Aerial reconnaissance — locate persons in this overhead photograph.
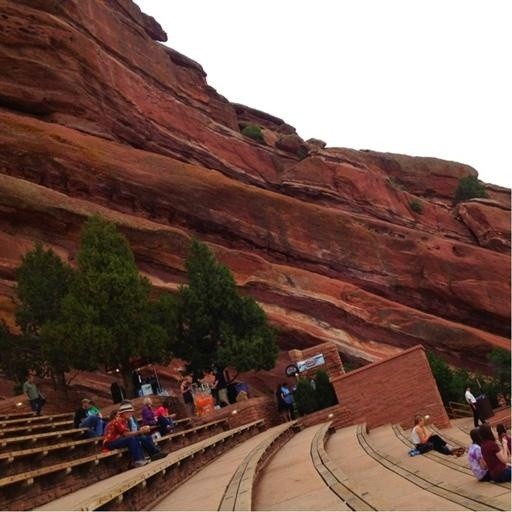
[181,378,194,417]
[22,374,46,417]
[464,386,489,428]
[412,416,512,484]
[281,382,298,421]
[73,396,178,468]
[275,383,295,423]
[211,366,231,407]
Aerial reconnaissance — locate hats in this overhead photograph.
[118,403,135,414]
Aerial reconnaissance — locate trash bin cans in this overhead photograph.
[110,382,122,404]
[475,394,494,420]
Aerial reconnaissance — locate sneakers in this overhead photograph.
[455,445,464,457]
[128,452,169,469]
